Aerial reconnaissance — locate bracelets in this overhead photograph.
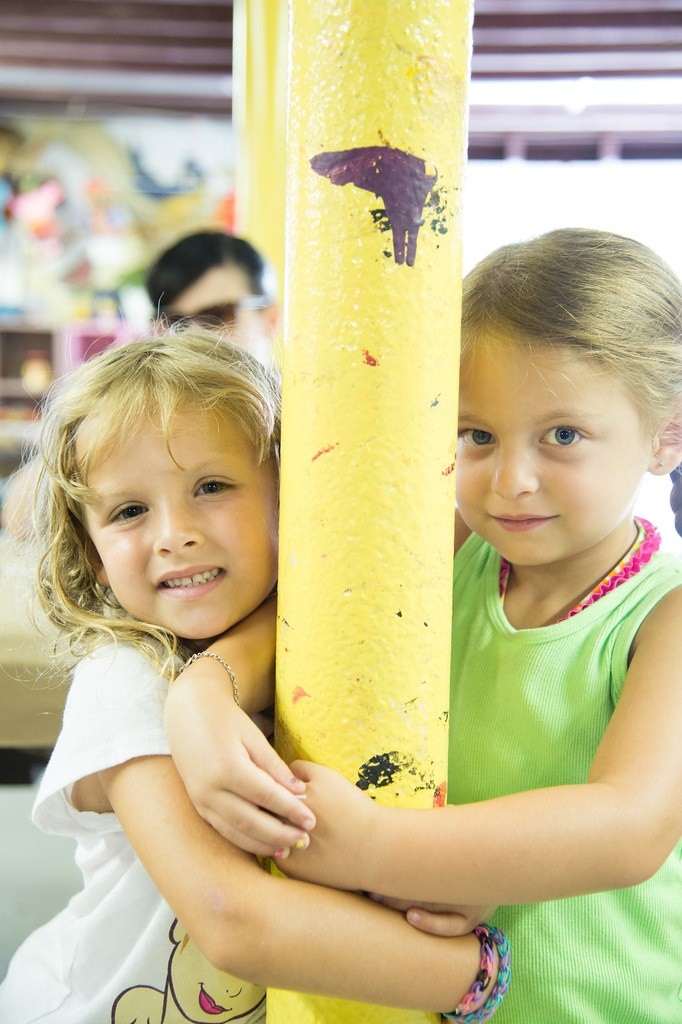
[443,924,511,1024]
[178,650,240,705]
[455,926,495,1015]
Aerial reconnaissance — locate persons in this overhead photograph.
[144,231,279,368]
[161,226,682,1024]
[1,327,512,1022]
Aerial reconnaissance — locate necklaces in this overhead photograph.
[499,516,663,622]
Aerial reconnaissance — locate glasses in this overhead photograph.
[160,296,269,333]
